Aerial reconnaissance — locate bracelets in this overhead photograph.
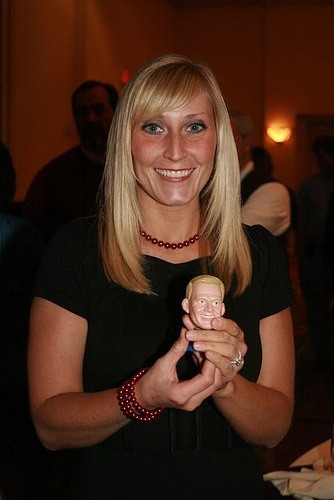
[118,367,166,422]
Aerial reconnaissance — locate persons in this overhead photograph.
[26,54,296,500]
[181,274,226,330]
[0,79,334,434]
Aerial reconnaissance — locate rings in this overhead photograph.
[230,350,244,366]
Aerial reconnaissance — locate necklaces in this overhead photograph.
[139,227,204,250]
